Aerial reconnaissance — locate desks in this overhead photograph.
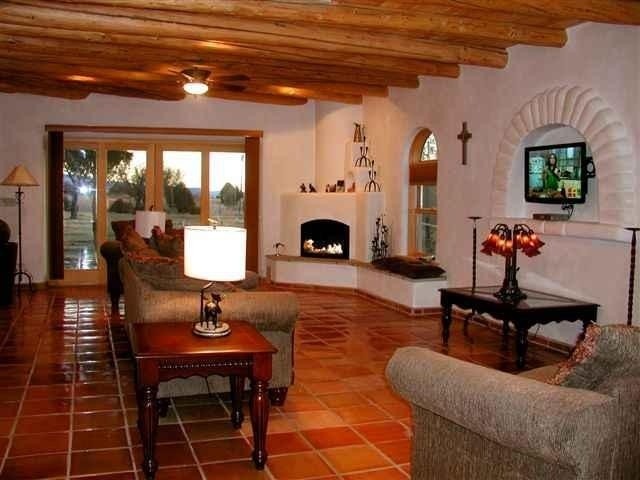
[125,319,279,479]
[436,284,601,372]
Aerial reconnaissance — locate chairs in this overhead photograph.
[119,256,303,418]
[382,341,638,480]
[98,238,153,317]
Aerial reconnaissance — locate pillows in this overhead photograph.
[540,319,640,402]
[111,219,239,293]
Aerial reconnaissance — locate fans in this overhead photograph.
[163,68,251,95]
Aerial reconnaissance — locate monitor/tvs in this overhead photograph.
[524,141,588,204]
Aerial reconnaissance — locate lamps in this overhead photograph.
[480,223,546,306]
[181,80,209,95]
[1,162,41,296]
[182,224,248,339]
[135,204,167,241]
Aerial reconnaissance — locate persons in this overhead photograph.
[539,152,563,199]
